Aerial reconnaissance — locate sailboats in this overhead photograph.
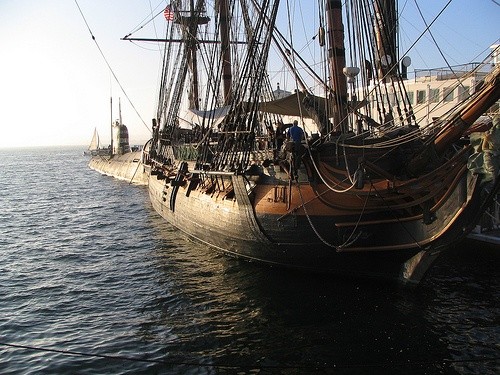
[83,128,103,156]
[121,0,500,281]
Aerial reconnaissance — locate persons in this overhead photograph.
[353,122,401,136]
[266,122,285,139]
[286,120,306,145]
[383,109,393,126]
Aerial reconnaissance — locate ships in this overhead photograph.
[88,97,150,185]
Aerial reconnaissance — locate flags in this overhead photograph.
[163,6,175,21]
